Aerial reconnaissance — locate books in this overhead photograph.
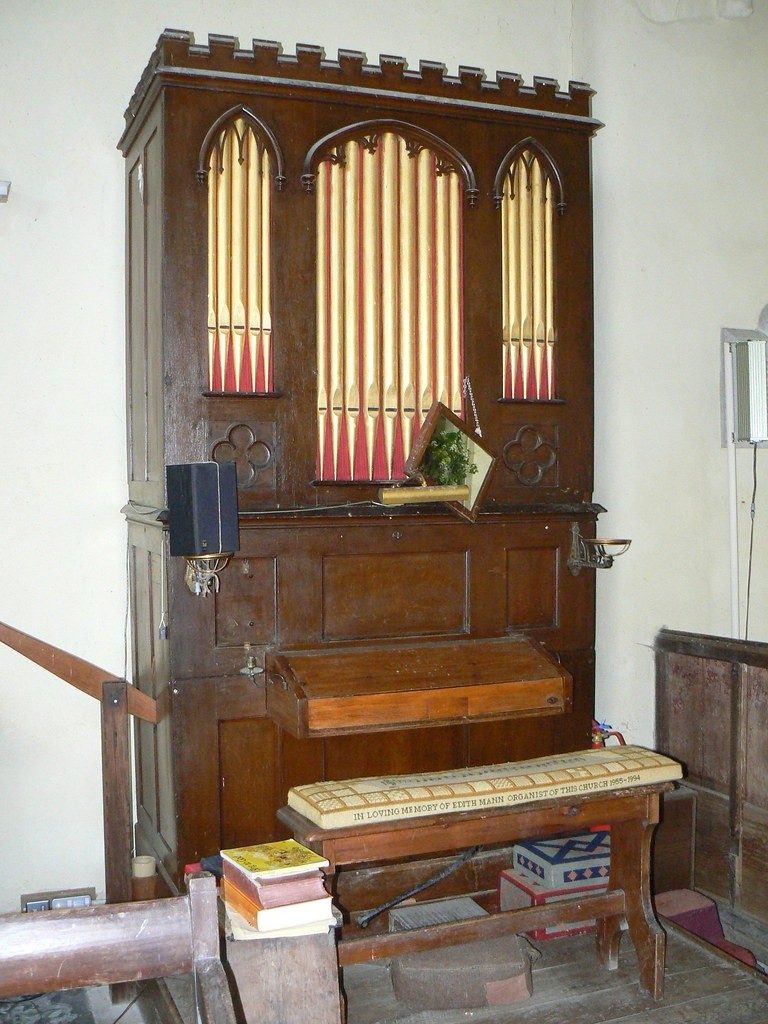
[184,837,338,941]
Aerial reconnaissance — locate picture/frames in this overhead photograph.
[402,401,501,524]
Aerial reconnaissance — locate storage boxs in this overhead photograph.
[497,869,609,940]
[513,831,611,889]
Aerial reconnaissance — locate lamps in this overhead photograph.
[378,472,469,505]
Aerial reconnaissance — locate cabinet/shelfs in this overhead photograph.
[116,25,604,942]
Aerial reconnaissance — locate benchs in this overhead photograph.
[276,745,683,1024]
